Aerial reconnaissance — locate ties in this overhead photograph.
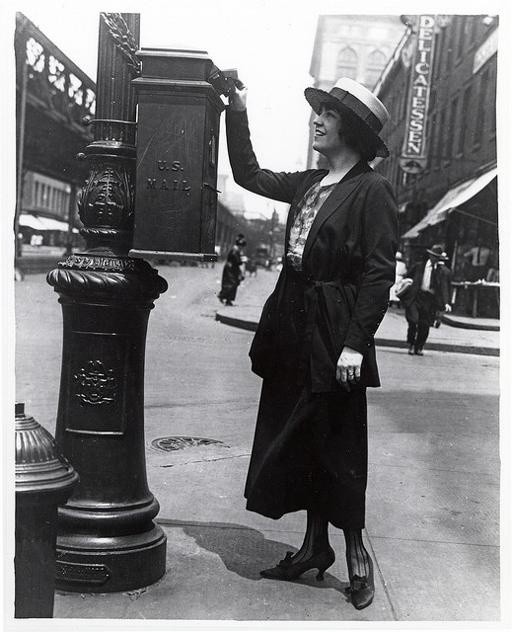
[430,263,437,288]
[477,246,481,264]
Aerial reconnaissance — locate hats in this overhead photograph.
[304,77,390,158]
[426,245,446,257]
[395,251,402,259]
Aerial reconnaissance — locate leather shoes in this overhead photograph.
[408,344,424,356]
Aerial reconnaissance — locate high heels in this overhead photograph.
[259,545,335,581]
[346,546,375,610]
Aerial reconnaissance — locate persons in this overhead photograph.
[224,76,400,610]
[401,245,452,356]
[430,252,452,328]
[388,252,407,308]
[217,234,247,306]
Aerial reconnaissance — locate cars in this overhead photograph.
[252,245,272,270]
